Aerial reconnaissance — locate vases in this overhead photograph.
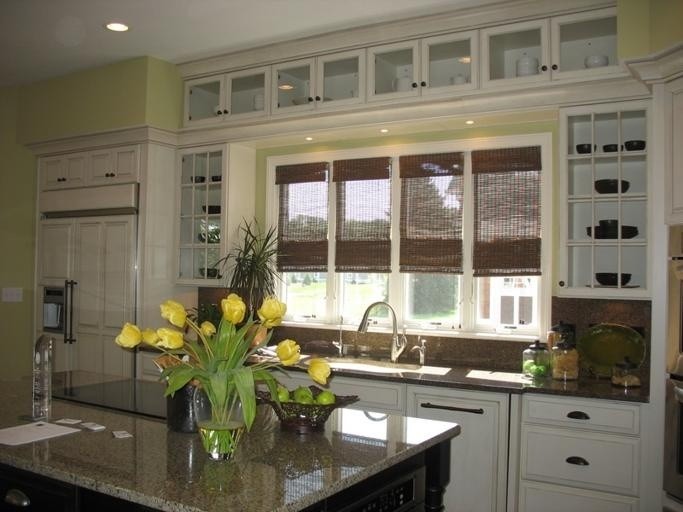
[193,384,251,462]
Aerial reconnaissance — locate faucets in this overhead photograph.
[358,302,407,363]
[411,340,426,365]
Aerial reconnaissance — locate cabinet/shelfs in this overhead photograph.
[254,367,509,512]
[520,393,662,512]
[36,146,140,190]
[560,100,653,300]
[35,214,139,411]
[182,7,620,130]
[175,144,255,287]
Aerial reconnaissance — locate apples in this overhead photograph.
[271,386,337,408]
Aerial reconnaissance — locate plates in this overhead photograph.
[586,226,636,238]
[254,94,263,107]
[586,283,639,290]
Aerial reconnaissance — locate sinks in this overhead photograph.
[304,357,422,373]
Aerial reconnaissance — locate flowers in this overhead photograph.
[116,292,331,453]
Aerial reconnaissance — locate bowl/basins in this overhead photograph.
[603,143,622,152]
[210,174,223,182]
[202,205,223,217]
[190,177,206,182]
[595,272,632,288]
[575,143,596,153]
[597,219,617,227]
[595,178,630,193]
[625,140,646,150]
[584,55,608,70]
[516,56,539,76]
